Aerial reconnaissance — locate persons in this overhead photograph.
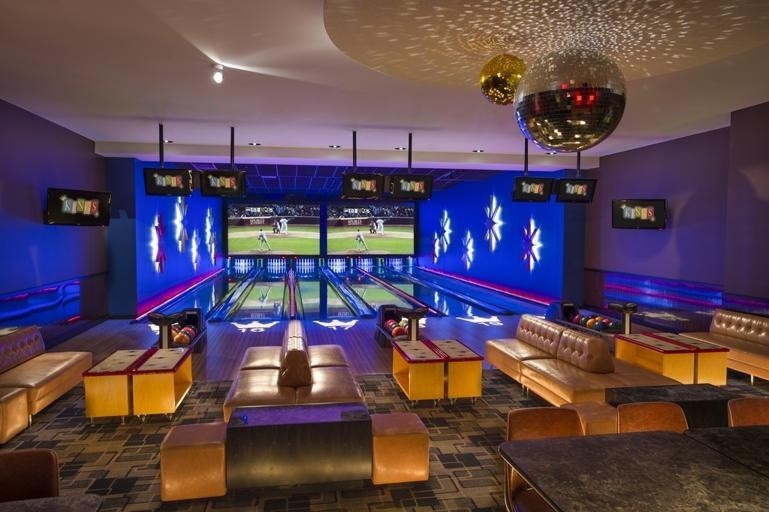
[258,228,272,250]
[272,218,288,236]
[355,229,368,251]
[369,218,384,236]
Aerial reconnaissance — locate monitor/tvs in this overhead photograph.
[389,174,433,201]
[513,176,553,203]
[328,199,415,255]
[557,179,596,202]
[224,201,321,257]
[341,173,382,199]
[144,167,191,196]
[200,169,245,197]
[46,188,111,226]
[611,198,665,229]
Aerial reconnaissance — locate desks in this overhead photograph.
[498,425,753,511]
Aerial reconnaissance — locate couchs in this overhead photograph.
[370,413,432,486]
[238,320,295,372]
[610,335,685,386]
[223,336,296,421]
[485,313,566,385]
[294,339,367,405]
[0,386,31,445]
[679,309,769,389]
[519,329,629,408]
[162,423,226,505]
[297,321,352,366]
[1,327,94,426]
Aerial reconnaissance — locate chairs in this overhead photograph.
[726,393,769,427]
[506,407,584,512]
[613,402,690,435]
[0,450,59,501]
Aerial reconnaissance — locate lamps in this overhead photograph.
[212,64,225,86]
[480,54,524,106]
[514,51,627,156]
